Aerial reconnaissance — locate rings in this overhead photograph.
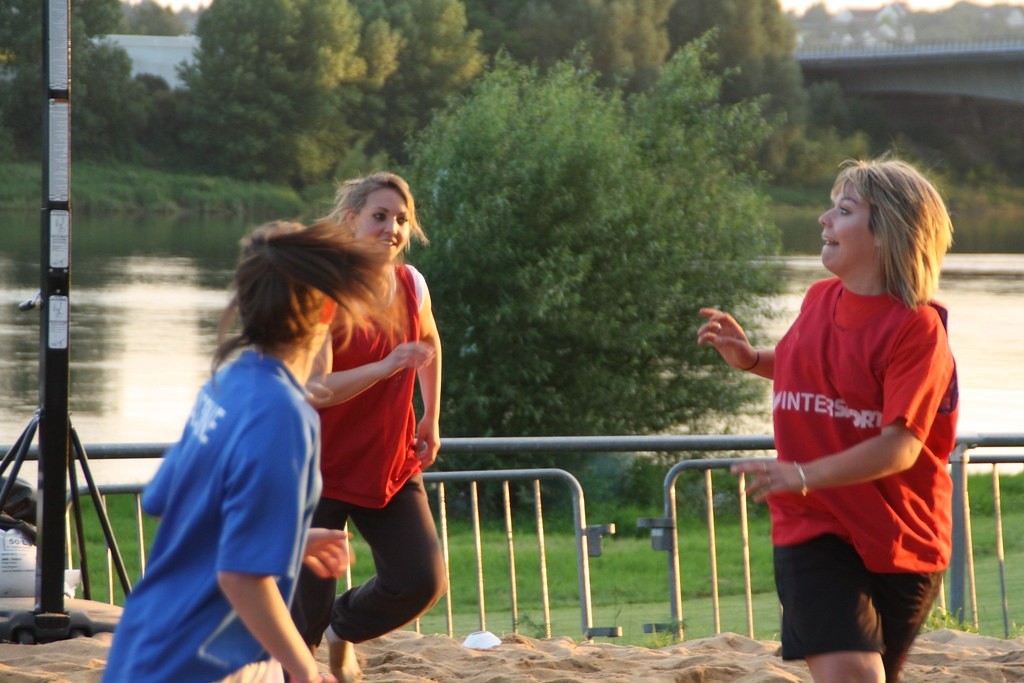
[763,462,768,472]
[766,474,771,486]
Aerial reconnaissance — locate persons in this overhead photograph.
[101,219,405,683]
[699,149,958,683]
[280,171,450,683]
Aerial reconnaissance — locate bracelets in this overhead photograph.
[792,459,807,498]
[741,351,760,371]
